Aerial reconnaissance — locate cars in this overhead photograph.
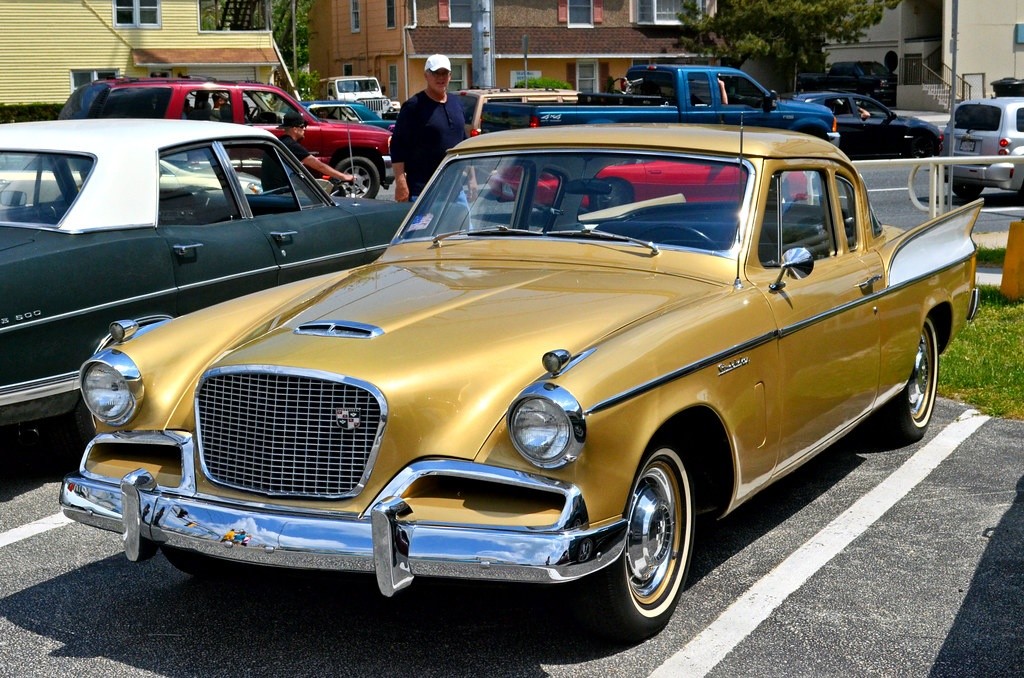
[0,152,263,213]
[781,87,944,158]
[0,118,478,466]
[58,123,986,653]
[275,98,399,130]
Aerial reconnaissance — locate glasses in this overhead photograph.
[295,125,306,129]
[429,69,449,77]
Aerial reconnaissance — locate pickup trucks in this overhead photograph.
[480,63,840,151]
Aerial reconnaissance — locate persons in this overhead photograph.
[261,112,358,195]
[717,78,728,105]
[212,93,250,124]
[845,99,870,119]
[390,54,478,231]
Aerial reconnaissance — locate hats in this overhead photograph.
[424,54,451,72]
[276,111,304,129]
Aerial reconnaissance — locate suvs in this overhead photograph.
[448,83,581,139]
[58,66,396,201]
[318,73,400,120]
[938,96,1024,205]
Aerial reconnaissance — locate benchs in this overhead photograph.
[594,201,849,262]
